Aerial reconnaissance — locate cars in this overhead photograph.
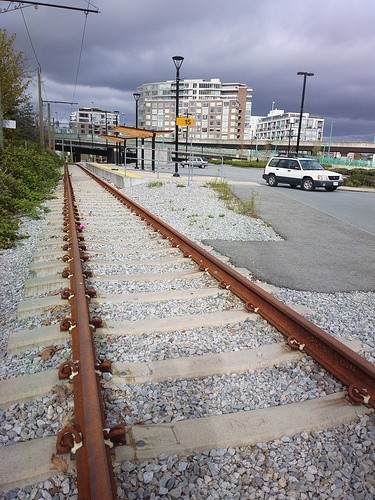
[261,156,345,192]
[180,156,209,169]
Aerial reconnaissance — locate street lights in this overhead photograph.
[133,93,142,170]
[114,110,121,165]
[91,101,95,142]
[172,55,186,176]
[122,113,125,126]
[296,71,315,153]
